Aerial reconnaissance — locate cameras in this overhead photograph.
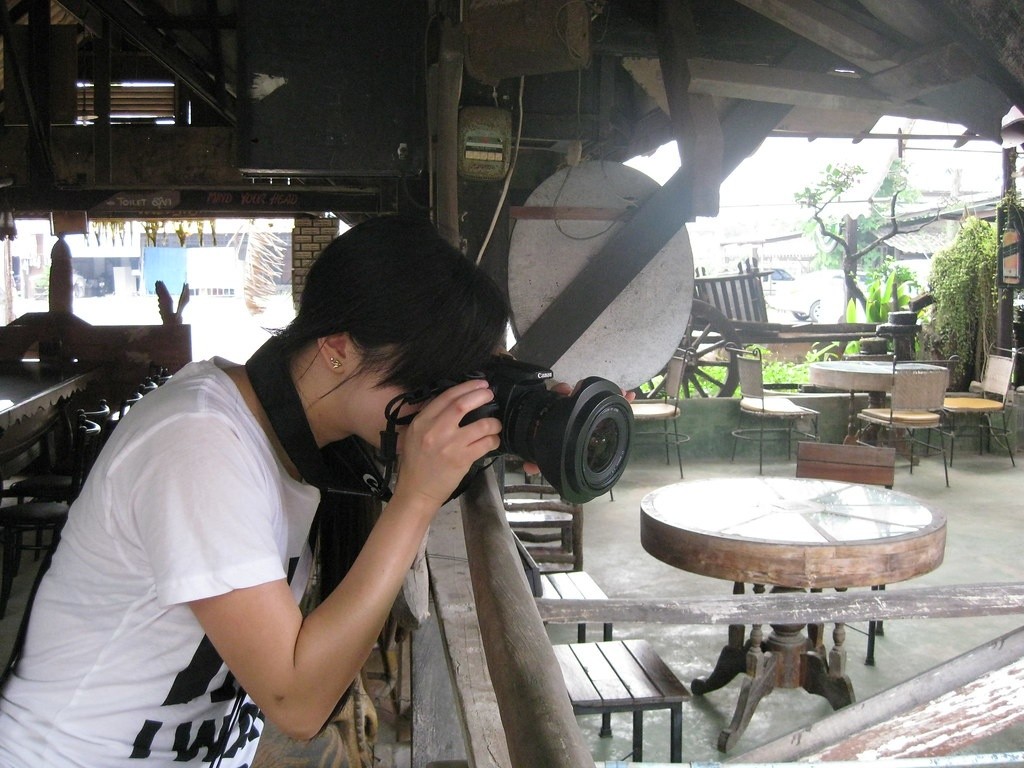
[423,355,635,507]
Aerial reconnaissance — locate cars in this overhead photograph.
[770,268,887,324]
[762,267,799,302]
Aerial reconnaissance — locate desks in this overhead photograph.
[1,358,100,431]
[638,478,945,752]
[808,360,934,446]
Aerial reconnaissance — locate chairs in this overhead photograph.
[1,364,173,621]
[924,342,1017,465]
[724,343,819,475]
[493,450,691,763]
[623,347,697,478]
[856,355,950,488]
[790,439,904,665]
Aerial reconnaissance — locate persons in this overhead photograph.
[1,217,637,768]
